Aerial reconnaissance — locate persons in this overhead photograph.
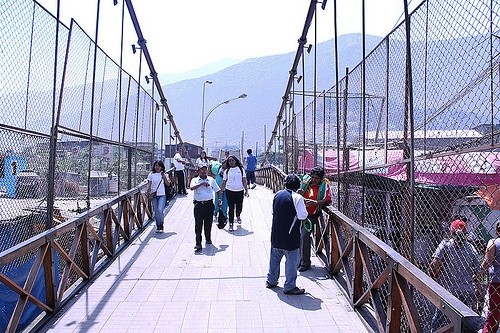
[245,149,257,189]
[190,164,227,250]
[220,155,249,231]
[195,151,235,222]
[172,147,189,195]
[145,160,171,233]
[266,173,310,294]
[296,166,332,272]
[356,210,500,333]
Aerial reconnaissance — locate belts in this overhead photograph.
[196,199,212,204]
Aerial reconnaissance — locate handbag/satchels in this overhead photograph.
[148,191,157,201]
[217,208,227,229]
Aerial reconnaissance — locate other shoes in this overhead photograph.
[178,191,182,195]
[251,184,256,189]
[206,239,212,244]
[229,223,233,231]
[183,191,188,195]
[297,260,311,272]
[283,287,305,295]
[247,185,250,189]
[266,280,279,288]
[194,245,202,249]
[237,217,242,224]
[155,226,163,233]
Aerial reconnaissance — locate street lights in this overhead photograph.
[202,94,248,150]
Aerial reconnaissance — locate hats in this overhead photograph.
[310,167,324,180]
[285,174,301,188]
[196,163,207,171]
[450,219,468,233]
